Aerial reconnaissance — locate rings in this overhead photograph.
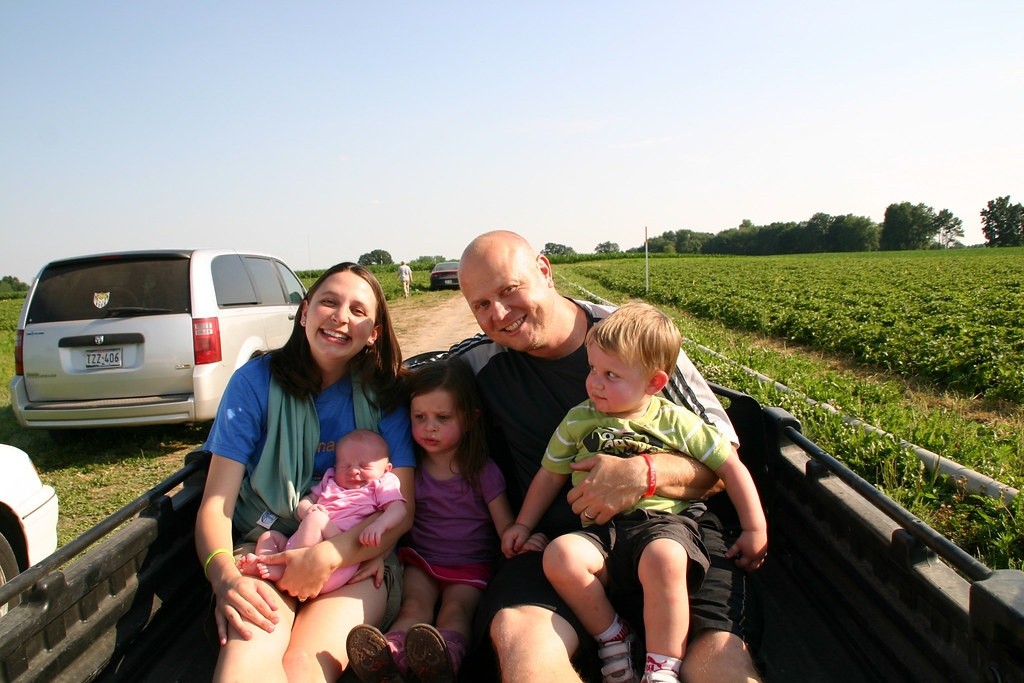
[584,510,594,521]
[298,596,307,602]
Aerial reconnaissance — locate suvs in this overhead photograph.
[9,246,310,437]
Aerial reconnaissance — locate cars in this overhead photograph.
[429,262,461,289]
[0,444,60,621]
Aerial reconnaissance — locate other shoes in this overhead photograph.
[404,623,453,683]
[346,624,409,683]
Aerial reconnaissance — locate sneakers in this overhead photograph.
[641,672,681,683]
[598,630,640,683]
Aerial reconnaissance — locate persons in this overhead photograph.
[194,262,415,683]
[397,261,412,298]
[501,303,768,683]
[402,229,763,683]
[236,430,408,595]
[346,358,548,683]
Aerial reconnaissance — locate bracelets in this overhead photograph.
[641,454,656,498]
[204,548,235,582]
